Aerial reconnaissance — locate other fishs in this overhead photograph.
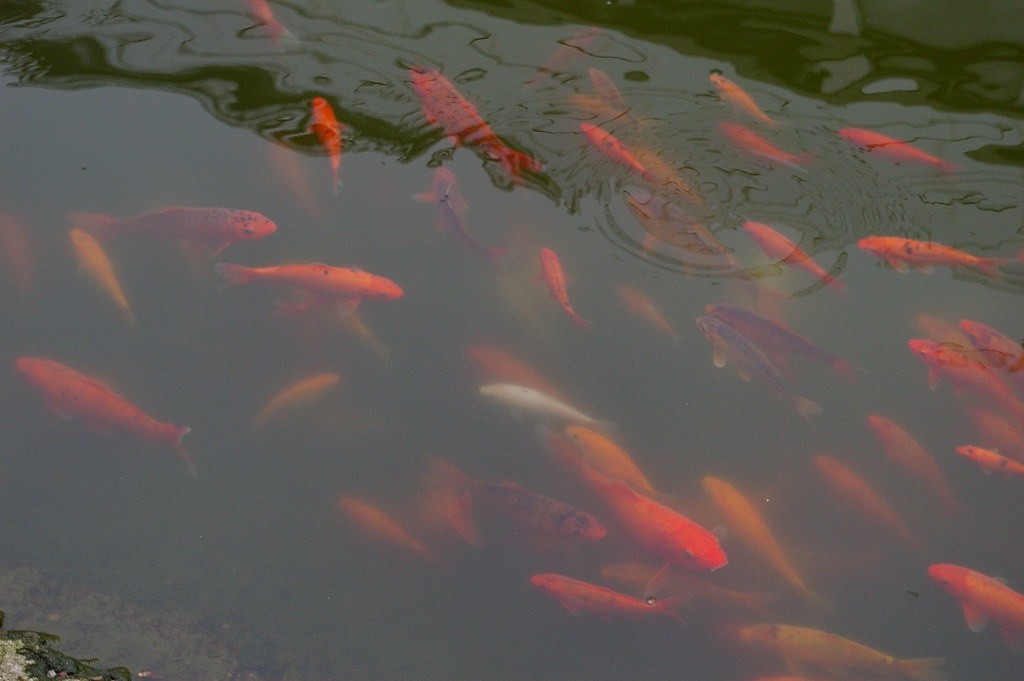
[1,2,1023,680]
